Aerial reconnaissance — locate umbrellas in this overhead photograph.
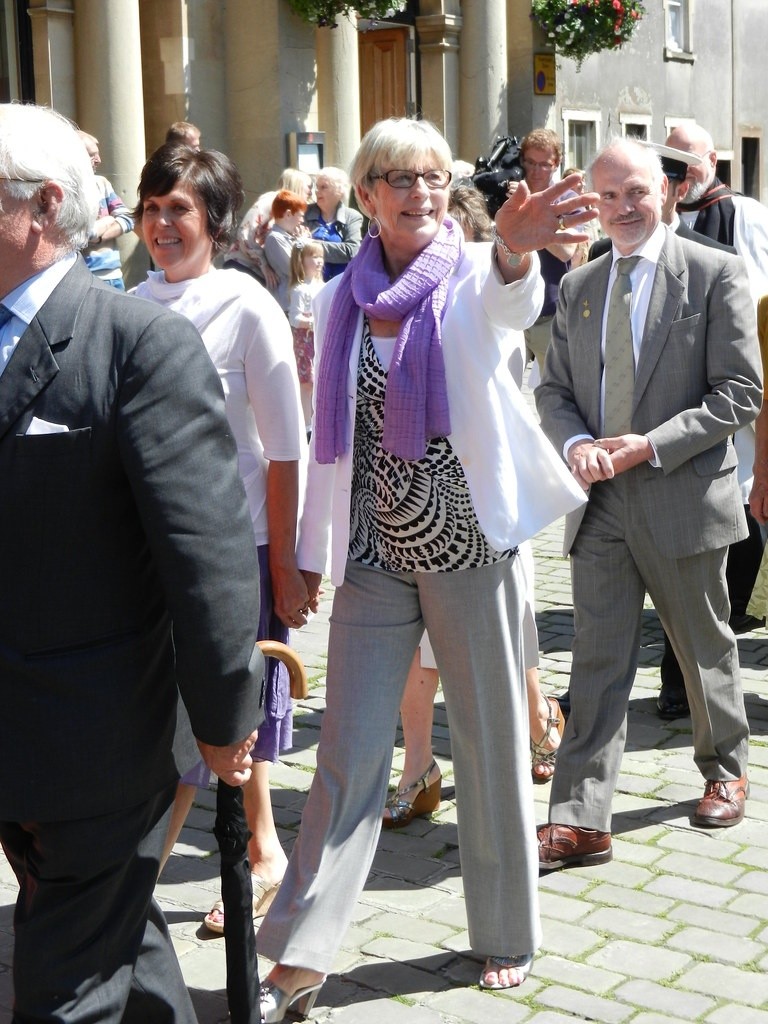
[213,637,307,1024]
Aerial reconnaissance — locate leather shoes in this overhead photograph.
[538,825,612,869]
[730,610,753,628]
[694,777,749,826]
[658,690,690,719]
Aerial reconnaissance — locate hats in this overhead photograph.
[639,140,704,181]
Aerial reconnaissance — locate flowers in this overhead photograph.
[530,0,645,74]
[286,0,408,36]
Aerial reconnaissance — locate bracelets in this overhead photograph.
[96,236,102,244]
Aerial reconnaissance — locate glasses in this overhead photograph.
[523,159,558,171]
[365,169,453,189]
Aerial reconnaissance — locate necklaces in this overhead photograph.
[490,226,529,269]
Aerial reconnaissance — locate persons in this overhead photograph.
[748,296,767,528]
[533,135,764,870]
[121,140,310,935]
[556,142,738,725]
[222,167,364,313]
[259,115,600,1024]
[447,160,609,269]
[132,121,202,242]
[665,122,768,629]
[380,184,564,831]
[507,127,591,388]
[288,242,328,444]
[0,102,266,1024]
[75,129,136,293]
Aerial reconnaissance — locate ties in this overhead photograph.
[604,257,643,439]
[0,304,15,329]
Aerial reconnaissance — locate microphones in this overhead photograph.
[501,154,516,169]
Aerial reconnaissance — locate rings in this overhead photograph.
[559,217,565,230]
[299,609,303,613]
[311,597,315,601]
[292,619,295,622]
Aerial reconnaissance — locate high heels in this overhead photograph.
[530,689,564,779]
[479,955,533,988]
[205,876,285,932]
[381,758,442,827]
[259,977,329,1024]
